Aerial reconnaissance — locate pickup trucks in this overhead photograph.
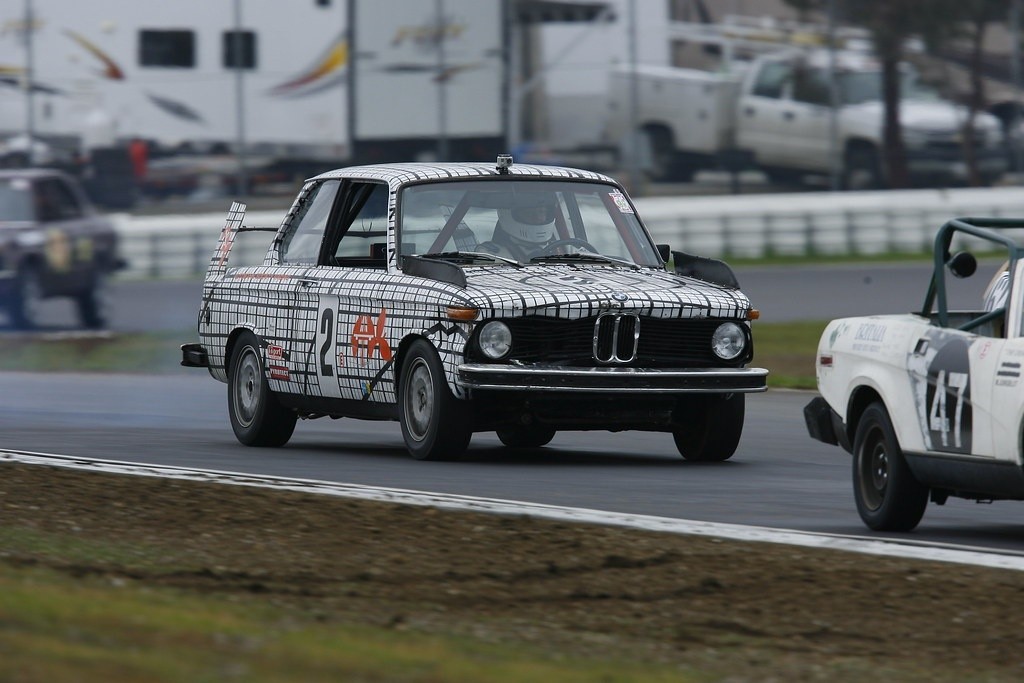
[603,50,1009,191]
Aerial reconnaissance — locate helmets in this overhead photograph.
[497,187,556,244]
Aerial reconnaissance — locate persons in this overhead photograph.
[476,191,591,261]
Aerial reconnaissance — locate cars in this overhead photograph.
[803,218,1024,530]
[0,170,128,328]
[180,155,769,461]
